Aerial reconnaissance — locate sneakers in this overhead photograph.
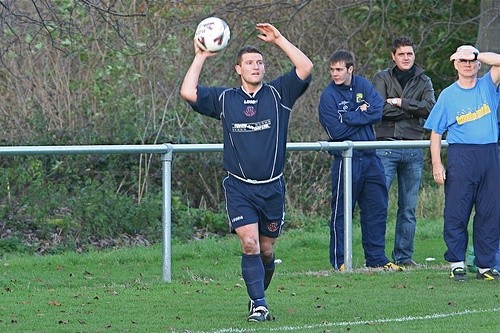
[449,267,467,281]
[383,262,402,272]
[477,269,500,280]
[248,300,255,315]
[248,306,269,322]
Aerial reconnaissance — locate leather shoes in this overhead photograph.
[405,260,427,267]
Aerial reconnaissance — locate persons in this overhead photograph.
[180,23,314,322]
[423,45,500,281]
[318,50,405,274]
[371,36,436,270]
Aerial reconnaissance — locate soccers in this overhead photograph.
[195,16,231,53]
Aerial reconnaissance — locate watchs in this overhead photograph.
[391,97,398,107]
[473,52,479,60]
